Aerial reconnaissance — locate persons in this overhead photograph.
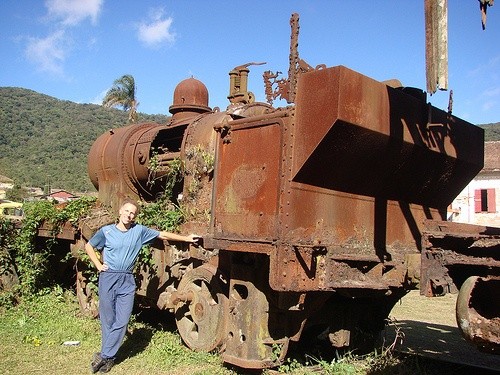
[85,203,203,374]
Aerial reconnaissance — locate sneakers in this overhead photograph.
[90,352,114,373]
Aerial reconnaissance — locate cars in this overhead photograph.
[0,201,23,221]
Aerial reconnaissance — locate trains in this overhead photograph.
[37,0,500,369]
